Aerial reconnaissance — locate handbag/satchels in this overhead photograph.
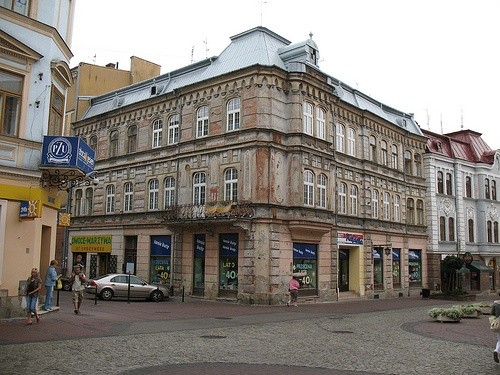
[55,278,62,289]
[488,315,500,332]
[21,295,27,309]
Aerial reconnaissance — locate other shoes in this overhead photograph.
[81,282,88,285]
[24,320,32,325]
[36,316,40,322]
[287,301,290,307]
[74,309,78,314]
[46,308,53,310]
[493,350,499,363]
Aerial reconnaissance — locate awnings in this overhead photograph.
[470,260,491,272]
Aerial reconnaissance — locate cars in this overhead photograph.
[84,273,169,303]
[54,276,73,292]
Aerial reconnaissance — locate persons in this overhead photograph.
[287,276,298,308]
[44,260,62,311]
[25,267,41,326]
[68,255,87,314]
[490,292,500,363]
